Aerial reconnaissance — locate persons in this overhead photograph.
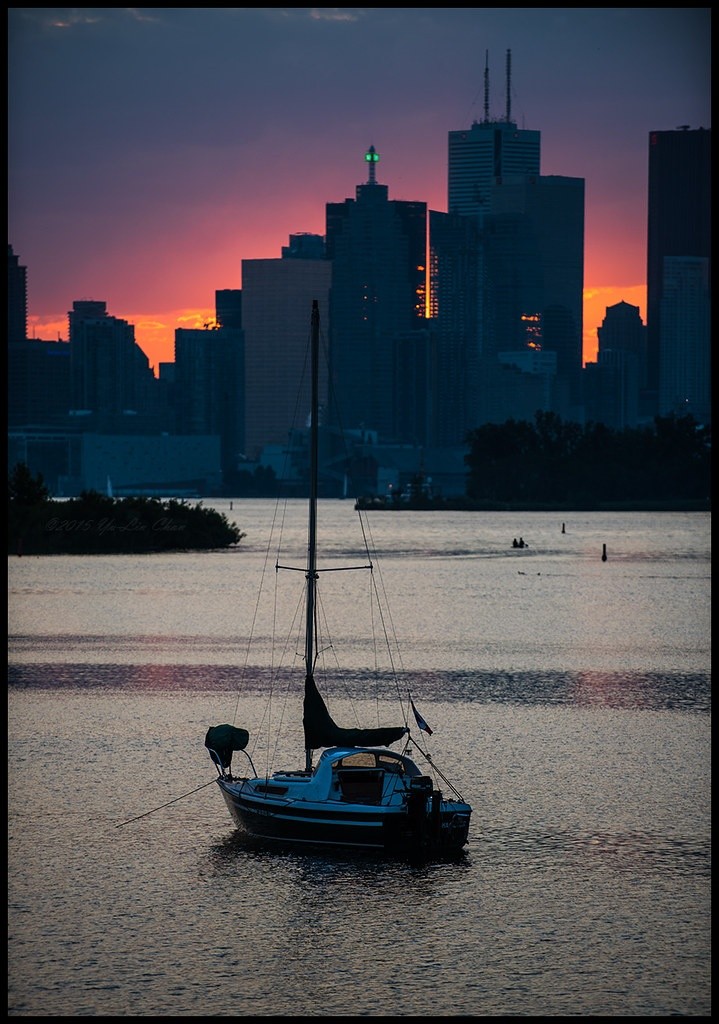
[519,537,525,548]
[513,539,519,548]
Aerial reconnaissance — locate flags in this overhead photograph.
[411,697,433,735]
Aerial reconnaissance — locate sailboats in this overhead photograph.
[201,300,474,855]
